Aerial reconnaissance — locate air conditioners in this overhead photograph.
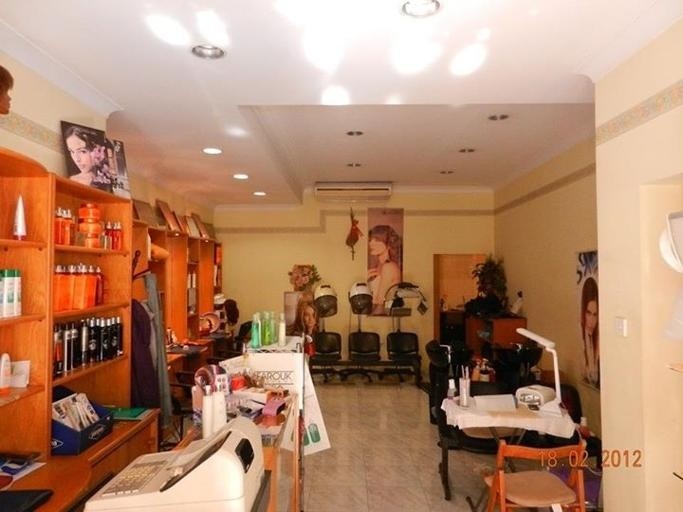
[313,181,392,201]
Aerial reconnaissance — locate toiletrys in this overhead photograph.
[250,311,287,350]
[201,382,228,439]
[0,353,11,399]
[55,194,127,398]
[1,268,23,318]
[12,194,28,239]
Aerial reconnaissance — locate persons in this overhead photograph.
[367,224,400,304]
[63,125,110,192]
[286,289,318,358]
[579,277,598,388]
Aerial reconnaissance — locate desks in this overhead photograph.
[443,394,566,512]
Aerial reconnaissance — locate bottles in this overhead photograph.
[471,358,497,383]
[200,382,227,440]
[250,309,287,350]
[0,202,124,378]
[579,417,591,439]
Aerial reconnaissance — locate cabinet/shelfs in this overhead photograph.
[464,312,526,348]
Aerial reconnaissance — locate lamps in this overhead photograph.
[515,327,562,414]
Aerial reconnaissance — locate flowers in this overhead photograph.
[286,263,322,293]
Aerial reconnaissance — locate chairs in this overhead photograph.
[308,331,422,382]
[483,439,586,512]
